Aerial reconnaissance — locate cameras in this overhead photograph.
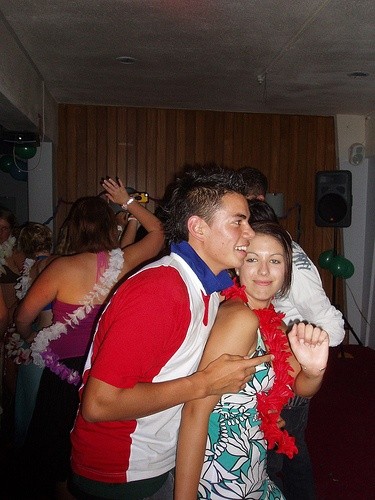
[131,192,149,202]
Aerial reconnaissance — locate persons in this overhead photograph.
[232,166,345,500]
[0,177,166,500]
[66,163,274,500]
[174,222,330,500]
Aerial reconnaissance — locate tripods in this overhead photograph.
[331,229,364,360]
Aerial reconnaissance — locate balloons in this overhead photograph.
[318,249,354,278]
[0,146,37,181]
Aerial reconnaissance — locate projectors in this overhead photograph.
[3,130,41,146]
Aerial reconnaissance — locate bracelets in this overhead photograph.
[300,364,327,371]
[117,225,122,231]
[121,197,134,210]
[128,215,138,221]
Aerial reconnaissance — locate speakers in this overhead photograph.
[348,143,364,166]
[315,170,352,228]
